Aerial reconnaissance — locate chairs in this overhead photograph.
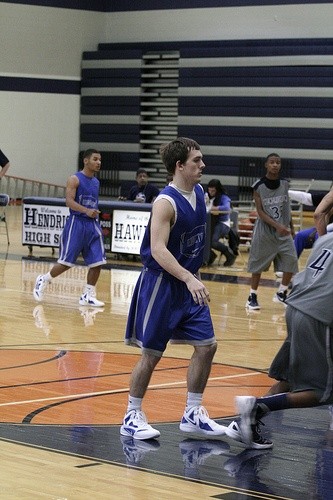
[217,209,247,266]
[0,194,11,245]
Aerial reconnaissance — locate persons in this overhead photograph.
[277,183,333,279]
[224,224,333,450]
[118,135,231,439]
[0,148,10,184]
[117,168,161,204]
[33,148,109,307]
[166,173,174,186]
[198,176,237,268]
[245,149,300,311]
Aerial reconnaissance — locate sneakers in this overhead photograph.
[246,293,261,310]
[272,288,289,307]
[33,274,47,302]
[119,409,161,439]
[235,396,266,445]
[224,419,274,448]
[179,404,228,436]
[79,291,105,306]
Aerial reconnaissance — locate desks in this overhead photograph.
[21,196,230,269]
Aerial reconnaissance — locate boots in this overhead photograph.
[221,247,237,267]
[208,250,217,265]
